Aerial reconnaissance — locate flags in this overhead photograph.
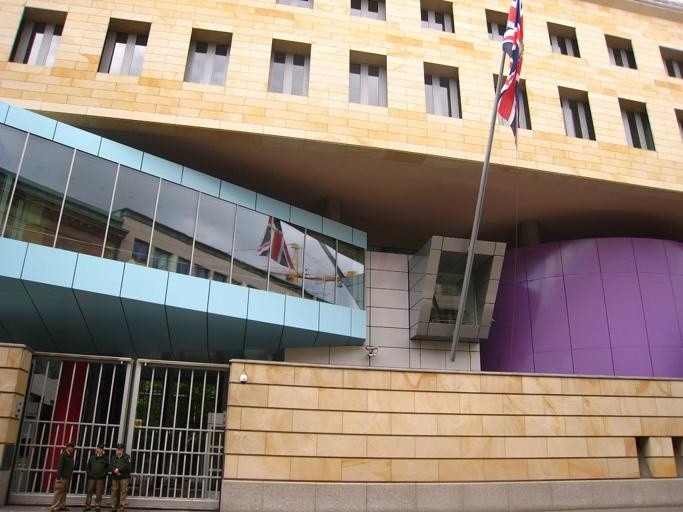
[495,0,524,153]
[256,216,296,270]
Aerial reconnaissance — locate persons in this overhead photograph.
[50,441,74,511]
[82,443,108,511]
[109,443,132,511]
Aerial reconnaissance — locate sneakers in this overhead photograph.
[52,507,101,512]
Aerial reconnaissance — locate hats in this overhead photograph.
[97,444,104,448]
[66,442,76,447]
[116,444,124,449]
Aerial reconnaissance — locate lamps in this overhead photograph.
[240,361,249,384]
